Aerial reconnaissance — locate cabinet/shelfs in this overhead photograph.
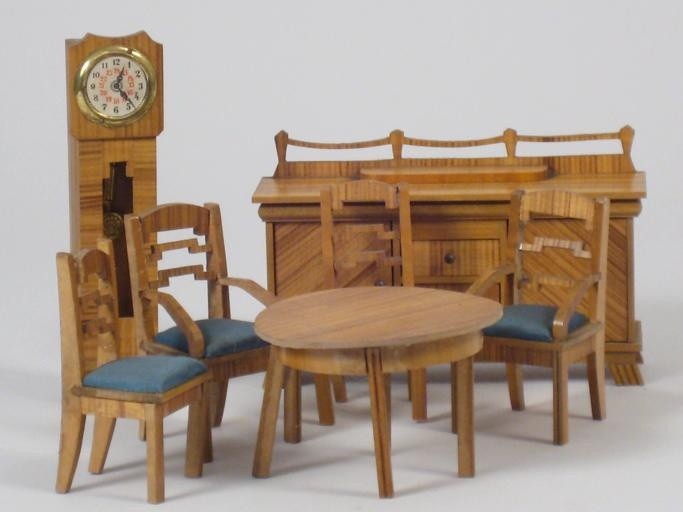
[252,124,647,383]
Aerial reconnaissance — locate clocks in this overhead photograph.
[63,33,163,375]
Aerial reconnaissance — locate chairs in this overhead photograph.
[314,182,428,426]
[57,239,211,503]
[449,189,610,443]
[121,202,300,468]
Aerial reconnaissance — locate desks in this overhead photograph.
[250,285,503,496]
